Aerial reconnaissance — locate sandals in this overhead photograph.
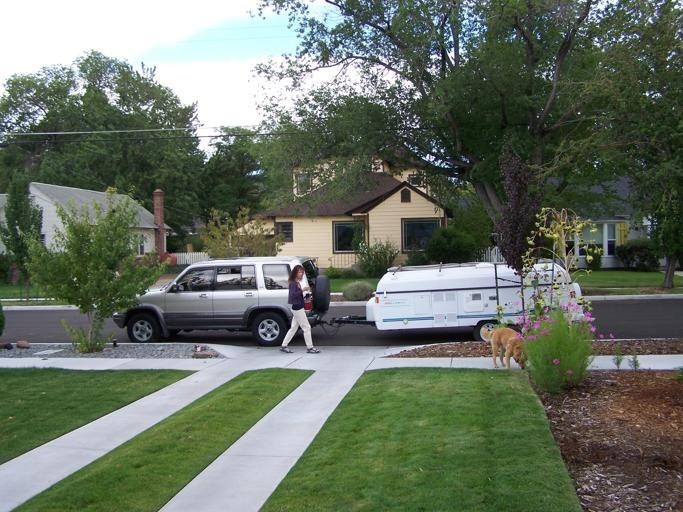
[280,345,294,353]
[307,347,319,352]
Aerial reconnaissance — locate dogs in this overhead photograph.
[480,327,527,370]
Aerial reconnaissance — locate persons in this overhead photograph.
[280,264,320,354]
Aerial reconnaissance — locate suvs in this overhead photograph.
[107,252,333,347]
